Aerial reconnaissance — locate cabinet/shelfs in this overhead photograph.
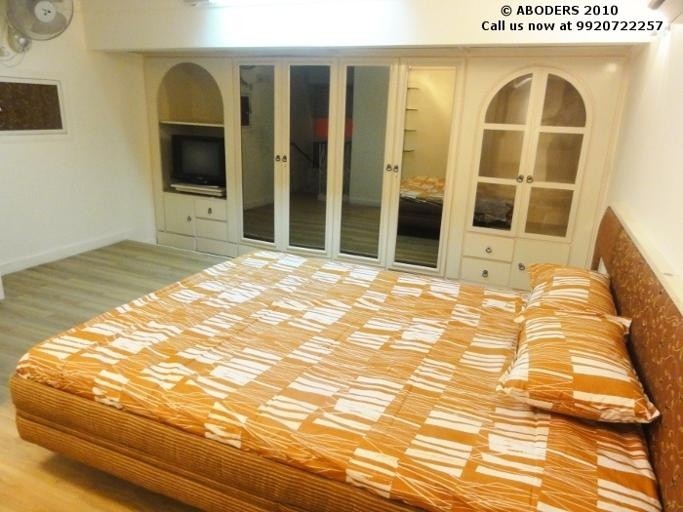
[445,46,627,293]
[404,83,420,153]
[155,63,238,258]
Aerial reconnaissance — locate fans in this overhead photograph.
[0,1,75,52]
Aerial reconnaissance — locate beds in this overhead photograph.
[7,205,682,511]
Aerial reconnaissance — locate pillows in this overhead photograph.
[497,261,660,430]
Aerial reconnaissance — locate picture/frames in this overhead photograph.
[0,76,67,135]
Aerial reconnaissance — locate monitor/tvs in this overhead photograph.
[172,134,225,188]
